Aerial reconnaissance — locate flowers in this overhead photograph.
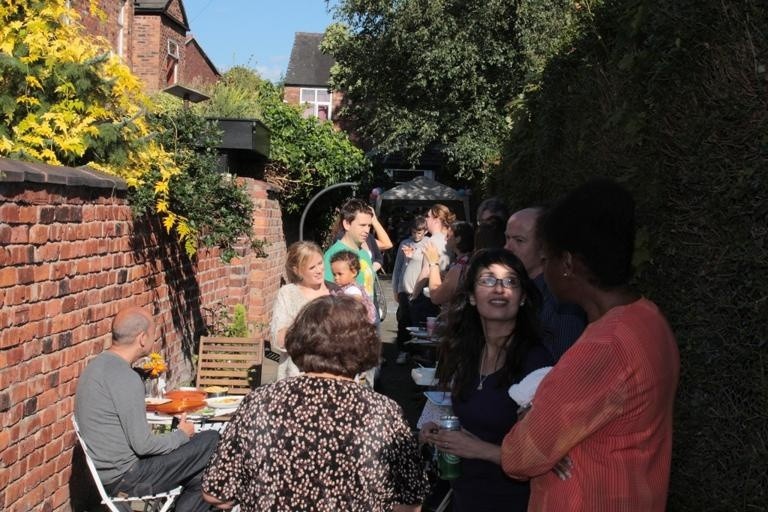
[143,354,168,379]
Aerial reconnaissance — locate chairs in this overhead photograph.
[70,415,183,512]
[195,335,264,394]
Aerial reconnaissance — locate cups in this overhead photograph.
[426,316,437,337]
[416,367,437,385]
[423,287,431,298]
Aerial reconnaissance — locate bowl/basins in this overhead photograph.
[208,392,227,398]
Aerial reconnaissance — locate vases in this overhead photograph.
[151,379,163,400]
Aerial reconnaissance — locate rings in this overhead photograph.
[445,442,449,449]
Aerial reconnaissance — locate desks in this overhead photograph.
[147,401,240,431]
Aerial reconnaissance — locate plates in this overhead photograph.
[204,395,245,409]
[405,326,427,337]
[423,391,453,407]
[145,396,173,406]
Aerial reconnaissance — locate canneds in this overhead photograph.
[438,416,461,482]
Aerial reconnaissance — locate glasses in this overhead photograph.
[475,278,521,289]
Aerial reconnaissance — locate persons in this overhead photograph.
[199,296,432,512]
[505,176,680,512]
[73,307,222,512]
[415,249,542,512]
[270,199,393,382]
[392,197,589,391]
